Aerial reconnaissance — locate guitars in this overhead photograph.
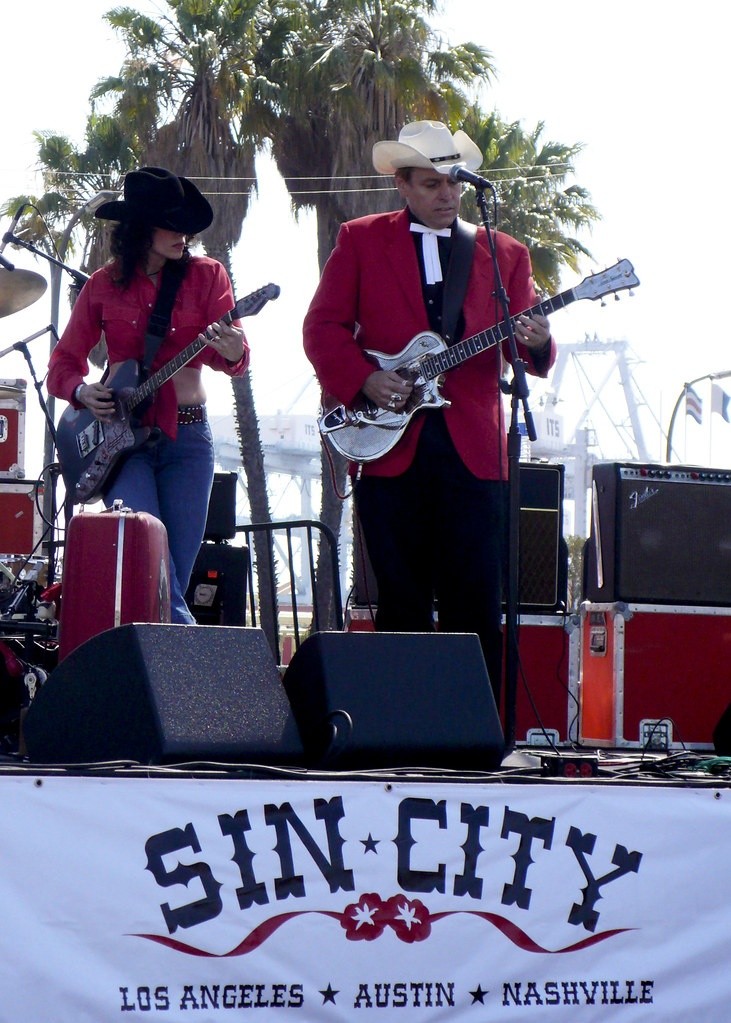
[55,283,281,504]
[318,257,640,464]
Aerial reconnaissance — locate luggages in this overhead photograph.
[56,496,169,667]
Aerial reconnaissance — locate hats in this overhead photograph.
[372,120,483,174]
[96,167,213,235]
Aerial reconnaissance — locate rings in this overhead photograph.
[219,332,228,338]
[214,336,221,342]
[391,393,401,401]
[388,401,395,408]
[402,380,408,386]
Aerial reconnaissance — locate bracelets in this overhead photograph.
[76,384,86,401]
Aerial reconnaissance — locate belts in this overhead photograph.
[178,408,203,424]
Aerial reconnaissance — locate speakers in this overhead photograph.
[184,472,248,627]
[19,622,303,764]
[589,463,731,606]
[353,459,565,608]
[282,632,505,770]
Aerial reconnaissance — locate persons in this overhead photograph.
[303,120,556,717]
[46,167,249,624]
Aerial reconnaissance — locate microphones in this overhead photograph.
[449,164,492,188]
[0,206,24,254]
[0,324,58,358]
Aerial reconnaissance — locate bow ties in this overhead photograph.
[410,222,452,284]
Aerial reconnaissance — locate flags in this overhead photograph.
[711,384,731,423]
[684,381,702,425]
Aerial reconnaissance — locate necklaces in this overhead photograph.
[145,263,165,277]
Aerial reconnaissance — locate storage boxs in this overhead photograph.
[576,600,731,751]
[345,607,574,747]
[0,378,27,479]
[0,483,45,556]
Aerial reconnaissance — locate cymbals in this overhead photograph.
[0,267,48,319]
